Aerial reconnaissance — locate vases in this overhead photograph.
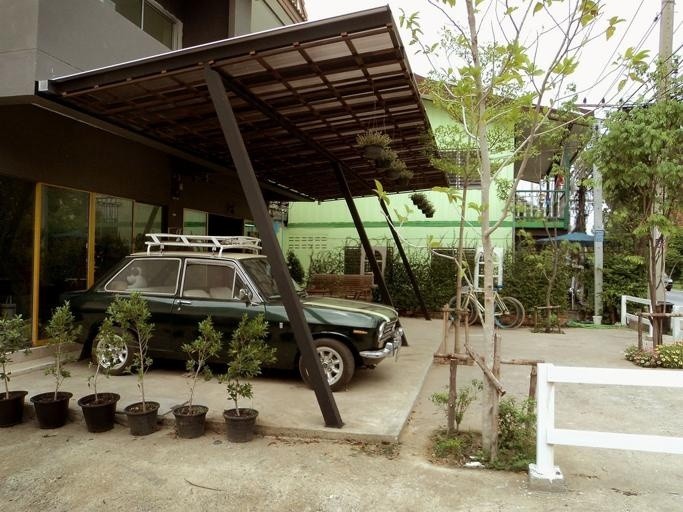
[592,315,604,326]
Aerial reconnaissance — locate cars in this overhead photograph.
[662,271,673,292]
[59,233,404,391]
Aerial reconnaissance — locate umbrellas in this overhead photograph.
[532,230,617,247]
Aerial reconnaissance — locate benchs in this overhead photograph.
[302,272,379,303]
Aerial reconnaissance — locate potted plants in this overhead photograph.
[29,299,85,430]
[216,311,280,445]
[76,294,127,435]
[355,126,435,218]
[171,310,224,440]
[0,312,34,428]
[106,284,163,437]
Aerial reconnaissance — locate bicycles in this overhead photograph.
[447,285,525,329]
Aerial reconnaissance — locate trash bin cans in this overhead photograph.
[655,301,674,334]
